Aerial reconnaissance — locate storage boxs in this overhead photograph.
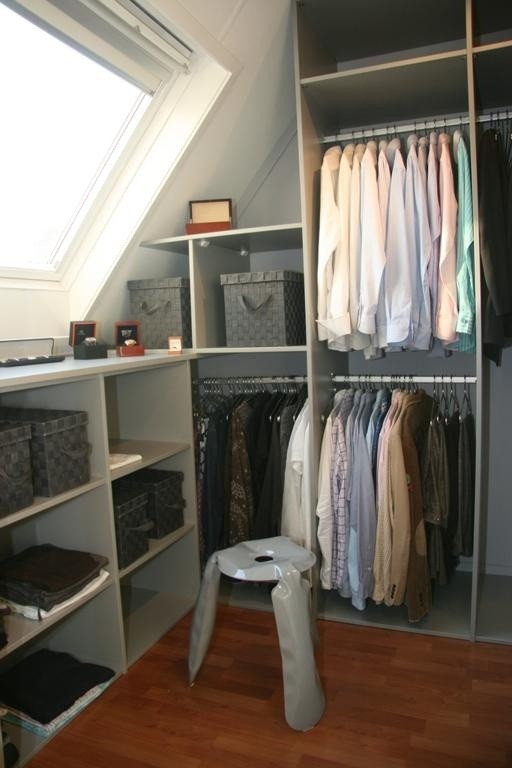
[3,404,91,498]
[111,486,150,572]
[218,270,304,347]
[124,467,187,540]
[1,422,34,518]
[127,277,189,350]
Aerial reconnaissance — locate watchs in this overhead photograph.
[82,337,97,347]
[124,339,136,346]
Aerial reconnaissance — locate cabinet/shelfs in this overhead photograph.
[1,350,201,743]
[140,0,512,646]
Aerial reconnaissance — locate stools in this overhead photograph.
[189,536,326,733]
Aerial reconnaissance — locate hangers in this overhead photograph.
[189,373,476,431]
[314,108,510,171]
